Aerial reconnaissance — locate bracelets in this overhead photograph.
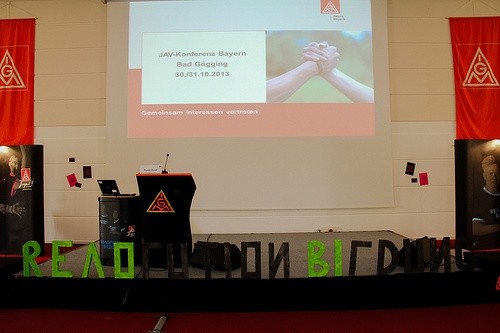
[314,61,322,75]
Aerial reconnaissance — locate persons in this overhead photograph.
[466,155,500,236]
[267,41,342,103]
[302,42,374,103]
[0,153,28,221]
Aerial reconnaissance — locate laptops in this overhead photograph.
[97,180,136,196]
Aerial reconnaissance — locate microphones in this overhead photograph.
[162,154,169,174]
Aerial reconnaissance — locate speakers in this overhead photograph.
[192,241,241,271]
[397,236,435,268]
